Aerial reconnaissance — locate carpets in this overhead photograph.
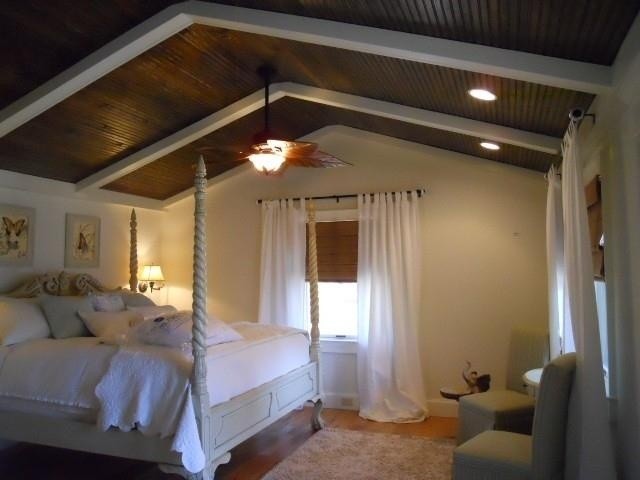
[261,425,459,479]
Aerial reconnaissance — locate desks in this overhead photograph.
[440,384,473,439]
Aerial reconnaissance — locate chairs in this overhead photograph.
[452,328,575,480]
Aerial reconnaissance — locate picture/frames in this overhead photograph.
[0,204,36,268]
[64,212,101,270]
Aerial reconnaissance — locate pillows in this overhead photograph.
[0,291,245,352]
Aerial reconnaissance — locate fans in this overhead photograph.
[187,66,355,170]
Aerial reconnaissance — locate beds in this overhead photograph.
[0,270,325,480]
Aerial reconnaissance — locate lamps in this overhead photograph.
[248,152,286,178]
[138,262,166,293]
